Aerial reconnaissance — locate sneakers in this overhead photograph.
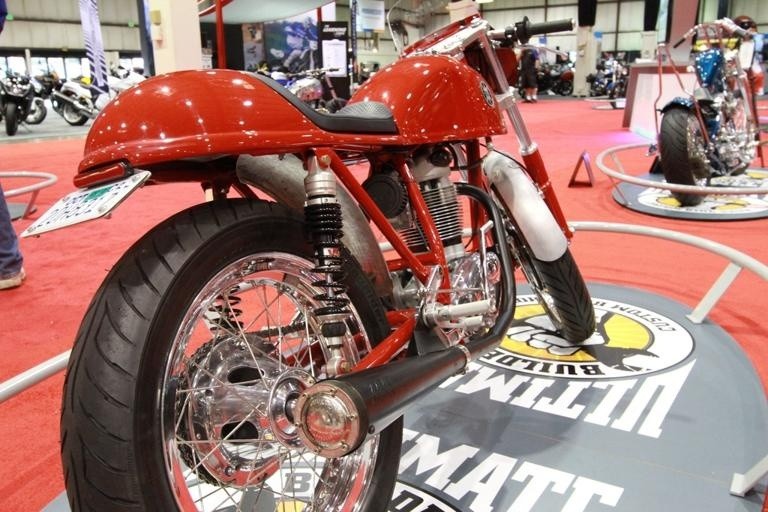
[0,267,26,290]
[523,98,536,103]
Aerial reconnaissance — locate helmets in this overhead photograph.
[734,15,757,42]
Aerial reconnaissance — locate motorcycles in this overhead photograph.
[589,50,631,99]
[654,18,765,205]
[17,11,596,512]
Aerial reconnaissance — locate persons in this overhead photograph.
[280,14,318,72]
[516,44,541,102]
[0,0,27,290]
